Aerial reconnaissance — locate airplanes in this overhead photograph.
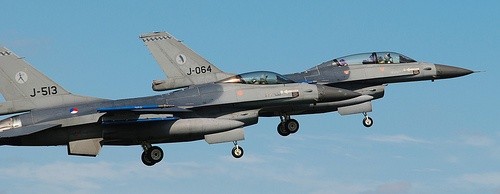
[0,30,483,165]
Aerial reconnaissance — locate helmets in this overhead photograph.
[344,64,348,66]
[387,53,390,55]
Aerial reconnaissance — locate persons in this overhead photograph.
[252,53,393,85]
